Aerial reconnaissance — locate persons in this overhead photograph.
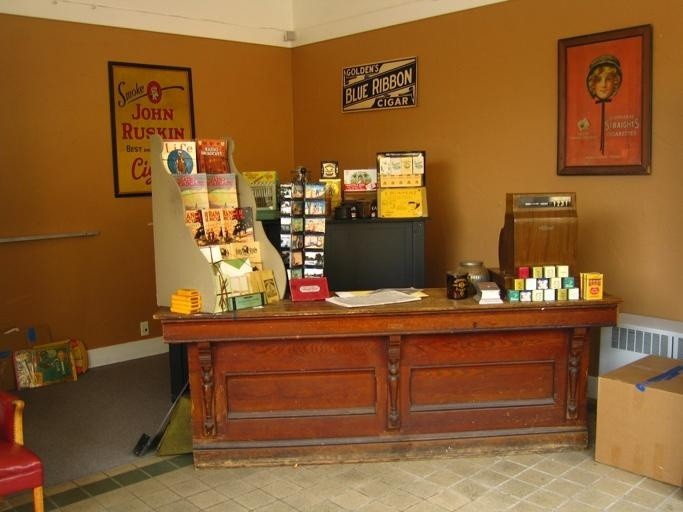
[585,54,623,101]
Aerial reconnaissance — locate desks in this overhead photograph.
[155,285,617,470]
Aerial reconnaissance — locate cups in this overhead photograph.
[447,271,468,300]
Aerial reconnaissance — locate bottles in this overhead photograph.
[456,261,490,296]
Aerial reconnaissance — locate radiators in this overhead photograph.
[599,311,683,374]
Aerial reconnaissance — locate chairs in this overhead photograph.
[0,390,44,511]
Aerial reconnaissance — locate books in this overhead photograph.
[163,137,280,313]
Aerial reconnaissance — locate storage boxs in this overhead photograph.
[593,354,683,488]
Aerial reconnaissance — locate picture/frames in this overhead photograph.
[106,61,196,199]
[555,23,652,176]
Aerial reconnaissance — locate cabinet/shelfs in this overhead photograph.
[149,133,286,313]
